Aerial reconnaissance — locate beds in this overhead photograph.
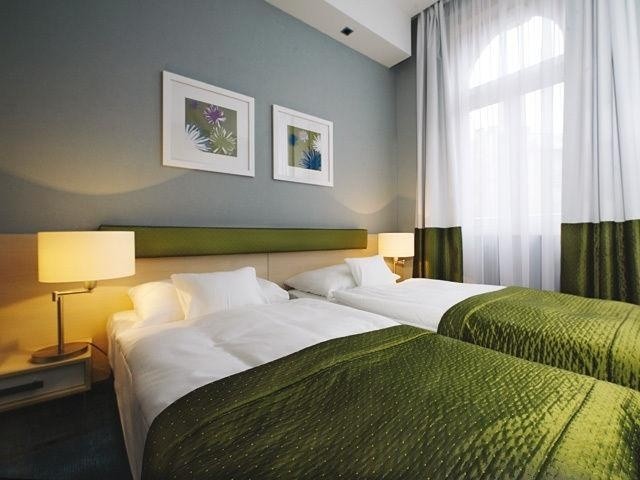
[288,277,638,389]
[106,295,639,479]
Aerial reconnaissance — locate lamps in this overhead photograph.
[28,230,138,366]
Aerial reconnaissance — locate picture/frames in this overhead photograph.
[269,102,336,189]
[158,66,256,177]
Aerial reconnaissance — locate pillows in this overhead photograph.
[126,271,286,330]
[171,265,269,320]
[344,255,397,287]
[282,264,402,297]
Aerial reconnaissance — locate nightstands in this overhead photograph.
[2,345,130,479]
